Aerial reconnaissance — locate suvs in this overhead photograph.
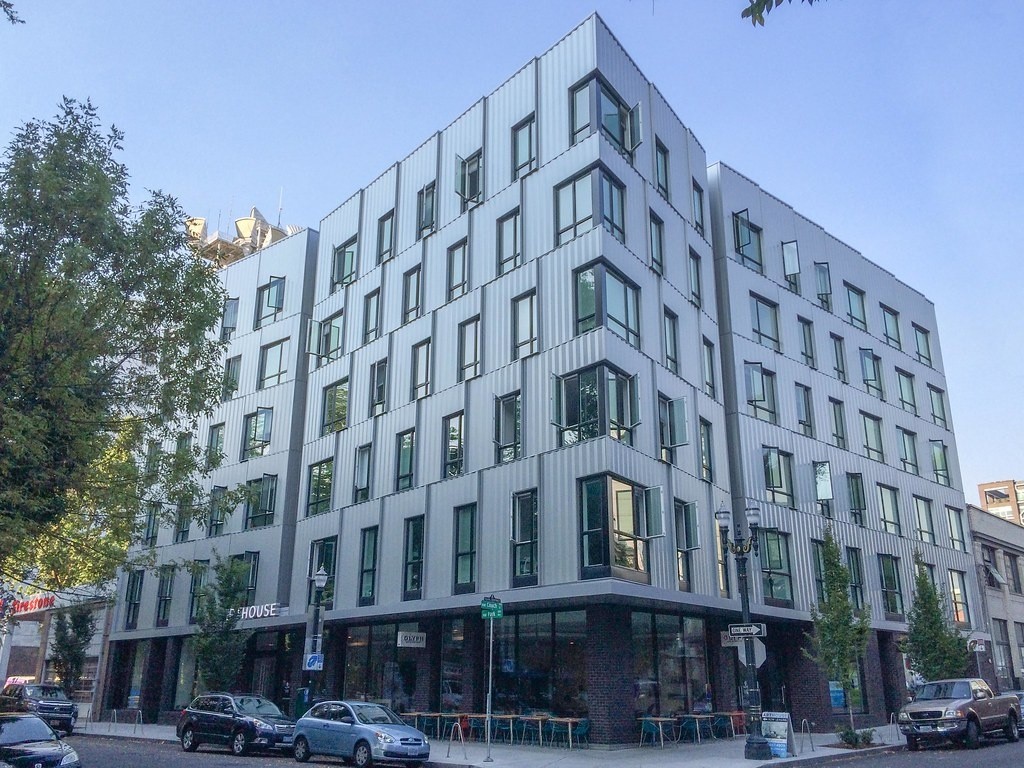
[176,691,298,756]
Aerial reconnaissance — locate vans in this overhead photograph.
[0,683,79,735]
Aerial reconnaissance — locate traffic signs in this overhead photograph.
[728,623,762,637]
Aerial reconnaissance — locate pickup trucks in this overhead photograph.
[897,678,1022,751]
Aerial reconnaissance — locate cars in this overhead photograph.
[0,712,82,768]
[292,701,430,768]
[982,690,1024,739]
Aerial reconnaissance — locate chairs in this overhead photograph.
[397,711,590,752]
[639,711,747,749]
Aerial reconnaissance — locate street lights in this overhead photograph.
[306,563,329,712]
[714,497,774,761]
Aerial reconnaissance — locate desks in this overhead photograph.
[637,717,677,750]
[400,713,429,728]
[442,714,470,742]
[493,714,520,745]
[420,713,449,741]
[677,714,717,745]
[549,718,583,751]
[467,714,494,743]
[520,715,549,747]
[710,712,747,741]
